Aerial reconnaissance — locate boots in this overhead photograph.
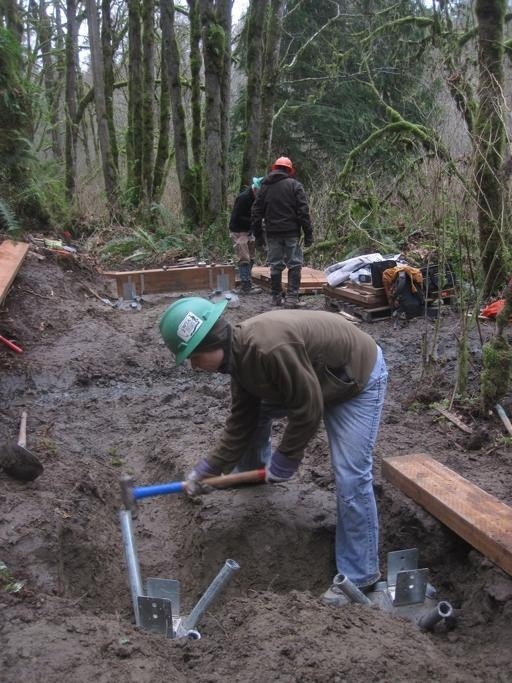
[270,273,284,305]
[239,260,263,294]
[285,267,307,309]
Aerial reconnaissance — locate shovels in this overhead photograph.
[0,409,44,484]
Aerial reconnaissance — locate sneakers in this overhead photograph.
[320,583,362,608]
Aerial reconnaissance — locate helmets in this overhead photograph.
[158,297,229,365]
[272,156,295,177]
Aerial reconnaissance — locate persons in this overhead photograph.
[250,154,315,309]
[226,175,268,294]
[159,293,389,603]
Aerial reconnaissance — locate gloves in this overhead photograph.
[303,232,313,247]
[184,469,215,498]
[255,235,266,251]
[264,462,291,484]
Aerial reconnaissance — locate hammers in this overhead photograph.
[119,469,266,521]
